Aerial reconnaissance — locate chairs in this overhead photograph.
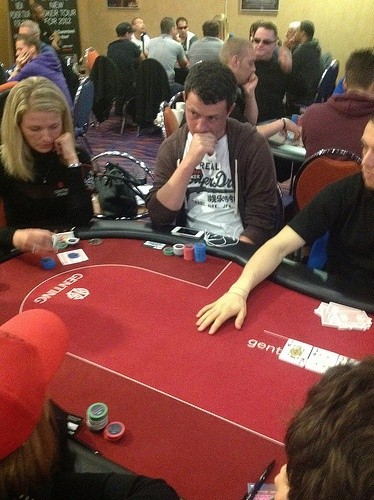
[89,149,155,219]
[71,78,97,154]
[158,89,187,139]
[291,149,365,263]
[286,59,341,113]
[134,58,172,138]
[84,46,134,135]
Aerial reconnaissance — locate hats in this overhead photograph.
[116,22,137,32]
[0,309,69,460]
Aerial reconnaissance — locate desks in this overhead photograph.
[253,118,306,173]
[0,220,374,500]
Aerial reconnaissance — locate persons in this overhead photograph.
[0,5,374,335]
[273,360,374,500]
[0,309,180,500]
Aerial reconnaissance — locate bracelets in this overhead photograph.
[228,286,248,299]
[14,66,20,72]
[282,118,286,129]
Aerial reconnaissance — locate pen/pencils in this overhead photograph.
[243,459,276,500]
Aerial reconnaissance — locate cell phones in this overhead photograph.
[170,226,206,240]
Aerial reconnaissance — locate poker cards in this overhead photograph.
[336,354,360,367]
[279,339,313,368]
[305,347,339,375]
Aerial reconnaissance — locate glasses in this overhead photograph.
[177,26,187,29]
[253,37,277,45]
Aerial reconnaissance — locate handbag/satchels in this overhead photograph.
[75,136,94,163]
[97,162,146,219]
